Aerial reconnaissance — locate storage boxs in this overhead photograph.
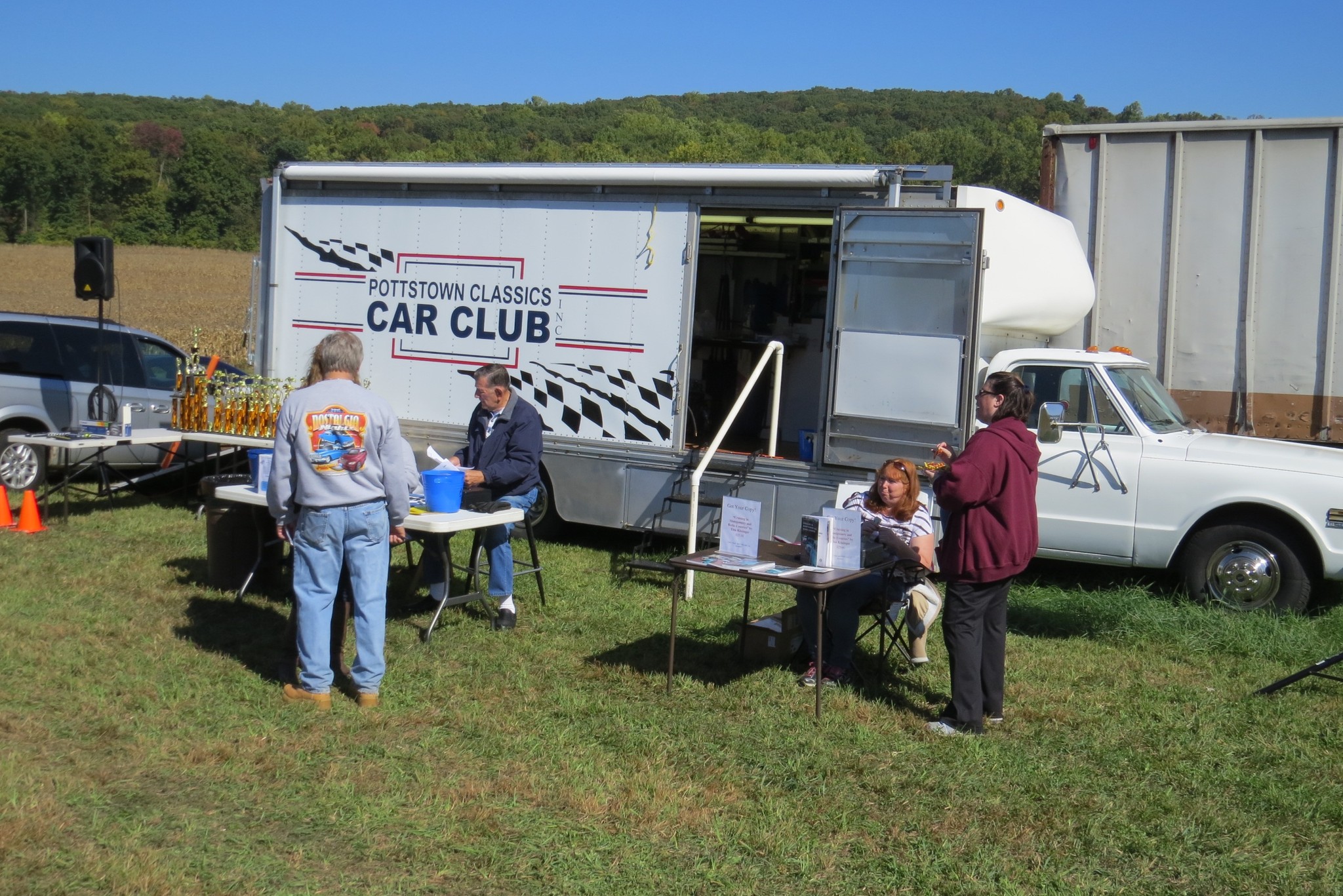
[79,420,112,436]
[462,486,491,508]
[860,541,883,567]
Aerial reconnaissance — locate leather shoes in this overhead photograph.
[405,594,439,616]
[496,607,517,631]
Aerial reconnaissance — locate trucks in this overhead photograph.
[242,164,1343,618]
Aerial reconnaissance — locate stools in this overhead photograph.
[438,467,546,627]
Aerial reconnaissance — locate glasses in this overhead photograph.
[884,459,909,479]
[978,388,1000,397]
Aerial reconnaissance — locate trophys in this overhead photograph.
[170,327,308,440]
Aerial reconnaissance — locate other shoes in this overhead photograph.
[282,684,332,710]
[360,692,380,711]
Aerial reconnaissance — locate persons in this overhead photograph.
[398,363,544,630]
[266,331,418,709]
[796,458,936,687]
[920,370,1040,735]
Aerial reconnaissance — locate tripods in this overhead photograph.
[8,301,168,508]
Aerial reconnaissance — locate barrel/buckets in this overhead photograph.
[422,471,465,514]
[247,449,274,489]
[799,429,818,463]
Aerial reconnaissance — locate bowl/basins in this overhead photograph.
[922,470,944,477]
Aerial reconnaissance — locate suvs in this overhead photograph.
[0,310,254,497]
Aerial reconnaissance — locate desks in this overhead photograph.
[181,432,274,507]
[666,537,897,723]
[216,484,525,643]
[695,335,811,437]
[7,425,195,527]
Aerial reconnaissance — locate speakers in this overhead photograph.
[74,236,114,301]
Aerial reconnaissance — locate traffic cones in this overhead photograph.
[0,485,18,528]
[8,490,49,534]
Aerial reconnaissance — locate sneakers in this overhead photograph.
[925,720,961,736]
[820,665,850,685]
[989,718,1002,725]
[800,662,822,688]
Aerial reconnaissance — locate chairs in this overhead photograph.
[801,560,925,682]
